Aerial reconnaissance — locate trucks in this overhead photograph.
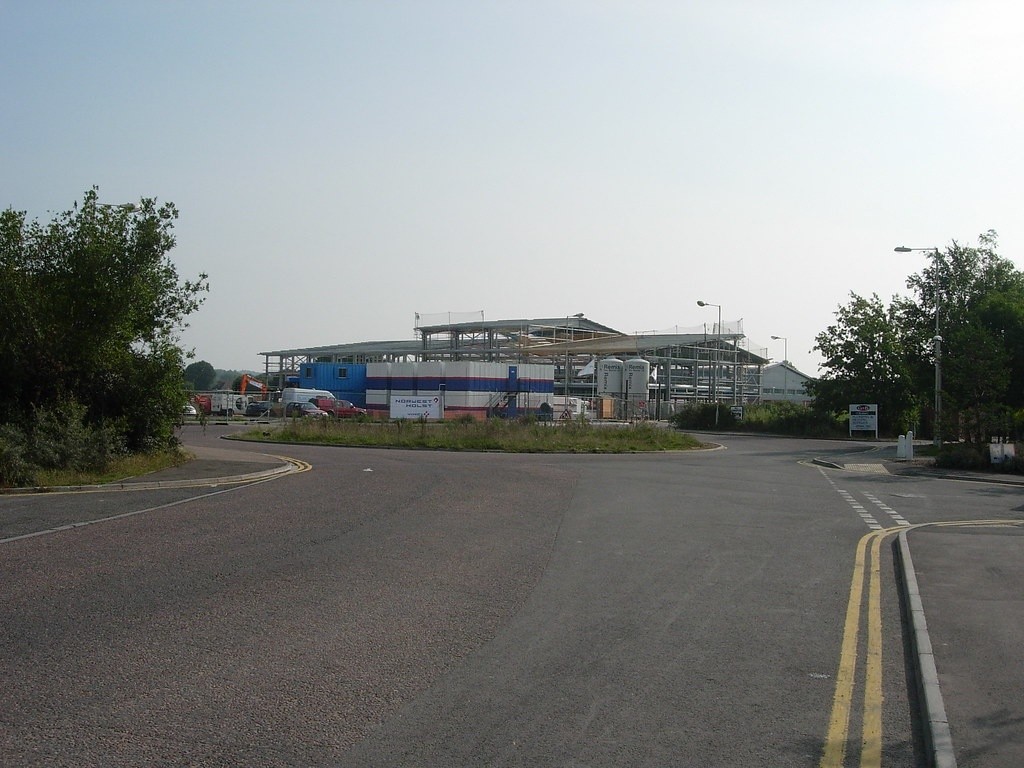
[195,392,256,418]
[308,397,368,417]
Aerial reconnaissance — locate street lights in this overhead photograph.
[772,335,788,410]
[895,245,944,458]
[697,300,721,430]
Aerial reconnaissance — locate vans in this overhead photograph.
[281,388,337,405]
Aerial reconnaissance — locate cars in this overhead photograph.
[245,401,274,417]
[286,402,329,419]
[183,405,197,416]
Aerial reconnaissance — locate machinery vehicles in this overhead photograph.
[239,373,268,398]
